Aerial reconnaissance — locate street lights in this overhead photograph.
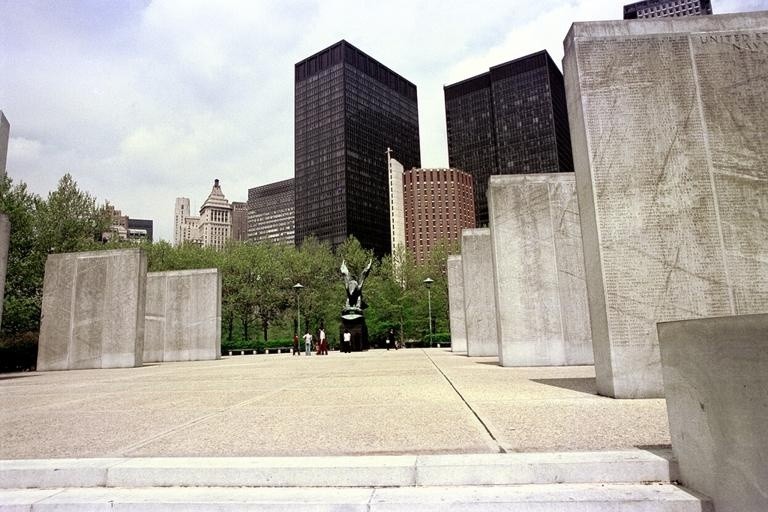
[293,283,305,349]
[423,278,436,346]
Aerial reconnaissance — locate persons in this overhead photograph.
[293,335,300,355]
[386,337,391,350]
[302,327,328,356]
[343,332,352,353]
